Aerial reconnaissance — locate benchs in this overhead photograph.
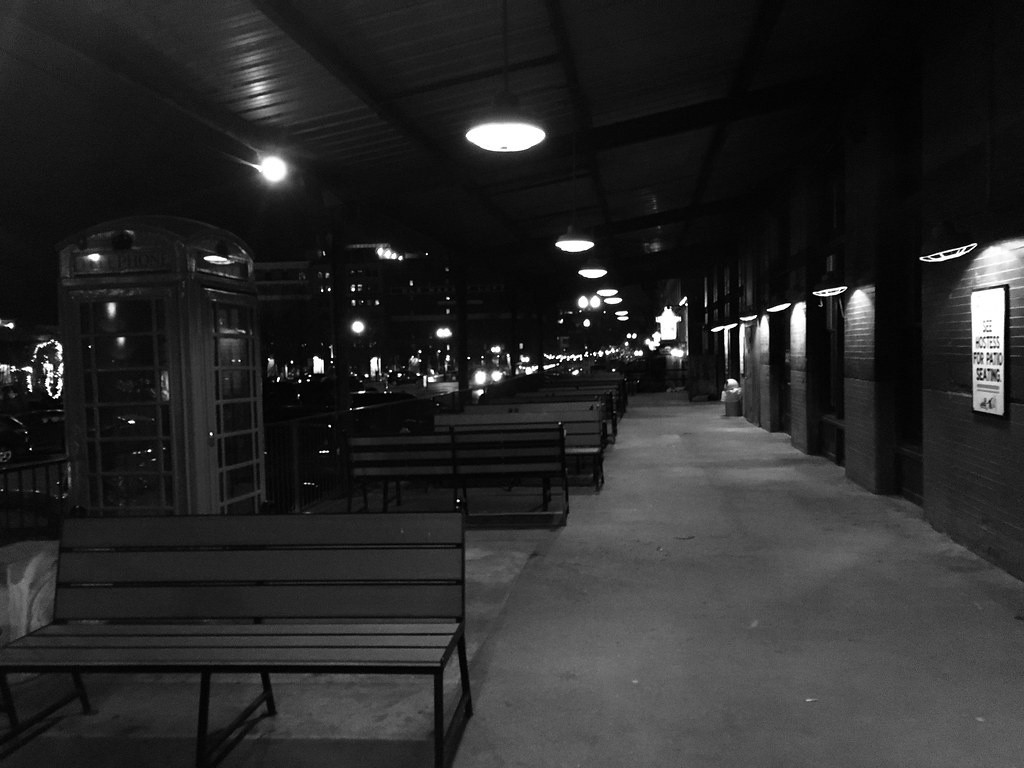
[0,496,478,767]
[341,374,629,529]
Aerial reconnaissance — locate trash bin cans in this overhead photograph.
[725,389,742,417]
